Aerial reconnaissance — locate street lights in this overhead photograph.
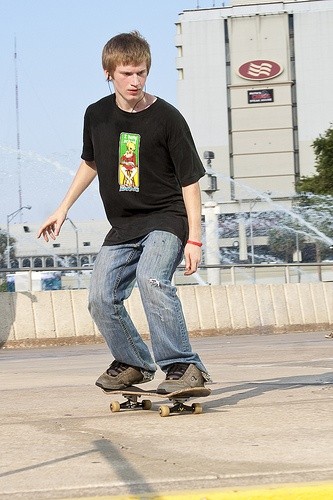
[65,218,81,266]
[7,206,32,270]
[250,189,274,284]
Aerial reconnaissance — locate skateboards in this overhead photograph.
[104,384,212,417]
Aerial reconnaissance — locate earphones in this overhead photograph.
[108,73,110,76]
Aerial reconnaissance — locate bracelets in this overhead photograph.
[187,240,202,248]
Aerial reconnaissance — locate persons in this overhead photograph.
[37,30,211,395]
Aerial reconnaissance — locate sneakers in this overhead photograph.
[95,360,154,390]
[156,362,204,393]
